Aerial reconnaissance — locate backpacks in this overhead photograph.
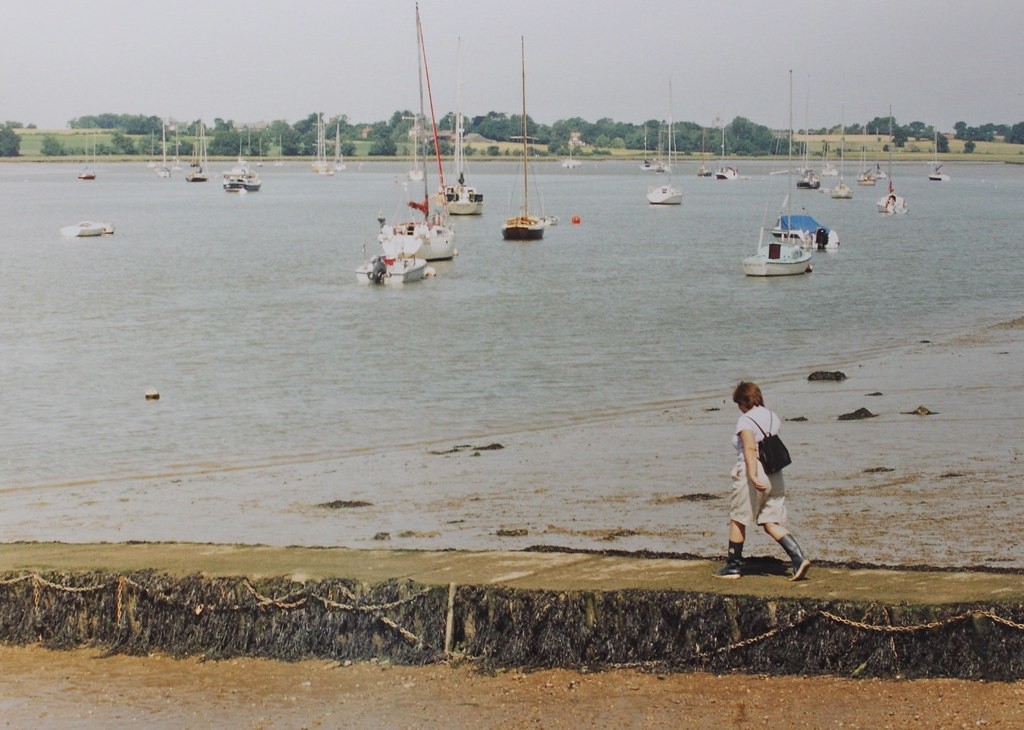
[745,410,792,474]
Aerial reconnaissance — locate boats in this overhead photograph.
[644,183,684,205]
[77,130,96,179]
[561,159,581,170]
[310,112,348,176]
[638,77,679,175]
[769,189,840,251]
[353,0,485,286]
[61,220,104,238]
[145,117,287,192]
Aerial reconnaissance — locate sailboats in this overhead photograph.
[716,116,739,180]
[739,67,817,278]
[829,104,855,200]
[875,104,908,216]
[796,70,889,190]
[695,126,712,177]
[927,122,950,182]
[499,34,548,241]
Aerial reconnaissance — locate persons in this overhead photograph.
[713,381,811,581]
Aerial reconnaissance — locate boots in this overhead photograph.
[710,540,743,579]
[777,534,810,582]
